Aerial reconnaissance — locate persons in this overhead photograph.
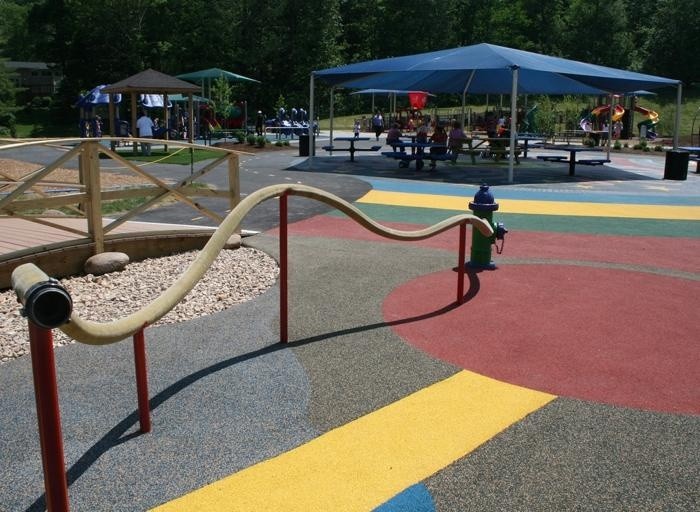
[386,122,405,158]
[407,118,414,131]
[353,121,361,138]
[601,123,609,146]
[498,120,520,146]
[487,115,505,162]
[83,107,194,156]
[447,121,467,166]
[253,104,320,139]
[615,119,623,139]
[416,126,428,153]
[373,110,385,140]
[428,120,447,168]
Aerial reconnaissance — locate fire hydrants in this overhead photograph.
[464,183,508,270]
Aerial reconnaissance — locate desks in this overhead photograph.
[479,134,546,161]
[677,147,700,174]
[334,138,370,162]
[400,135,431,155]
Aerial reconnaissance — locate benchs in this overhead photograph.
[322,142,611,176]
[689,157,700,162]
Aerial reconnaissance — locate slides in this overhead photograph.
[202,117,222,132]
[612,104,625,139]
[635,106,659,138]
[580,104,610,133]
